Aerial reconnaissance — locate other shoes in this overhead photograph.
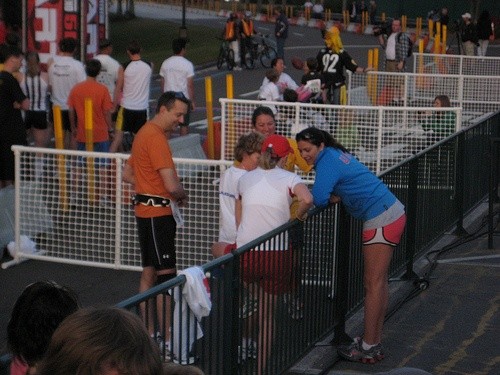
[289,300,305,320]
[241,340,258,359]
[240,300,258,318]
[70,194,86,209]
[233,67,243,70]
[98,195,115,210]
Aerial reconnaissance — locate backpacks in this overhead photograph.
[398,32,412,57]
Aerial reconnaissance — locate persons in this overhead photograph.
[237,10,257,69]
[347,0,378,25]
[475,9,495,63]
[219,11,240,72]
[427,8,449,35]
[255,26,375,150]
[459,14,478,69]
[374,18,411,102]
[420,95,457,139]
[4,280,205,375]
[211,134,315,374]
[295,127,407,362]
[219,130,265,359]
[304,0,325,20]
[121,92,197,364]
[251,106,317,320]
[0,37,201,208]
[273,6,288,68]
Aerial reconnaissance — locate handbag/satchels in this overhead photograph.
[490,33,495,41]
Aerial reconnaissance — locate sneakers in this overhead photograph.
[375,345,384,360]
[149,332,163,349]
[158,341,199,365]
[338,336,376,363]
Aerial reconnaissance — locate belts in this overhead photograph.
[387,59,400,62]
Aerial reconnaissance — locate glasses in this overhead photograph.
[174,92,185,99]
[296,134,314,139]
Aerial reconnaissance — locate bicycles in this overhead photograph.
[244,32,278,70]
[216,36,234,71]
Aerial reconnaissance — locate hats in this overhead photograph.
[461,13,471,19]
[261,135,294,158]
